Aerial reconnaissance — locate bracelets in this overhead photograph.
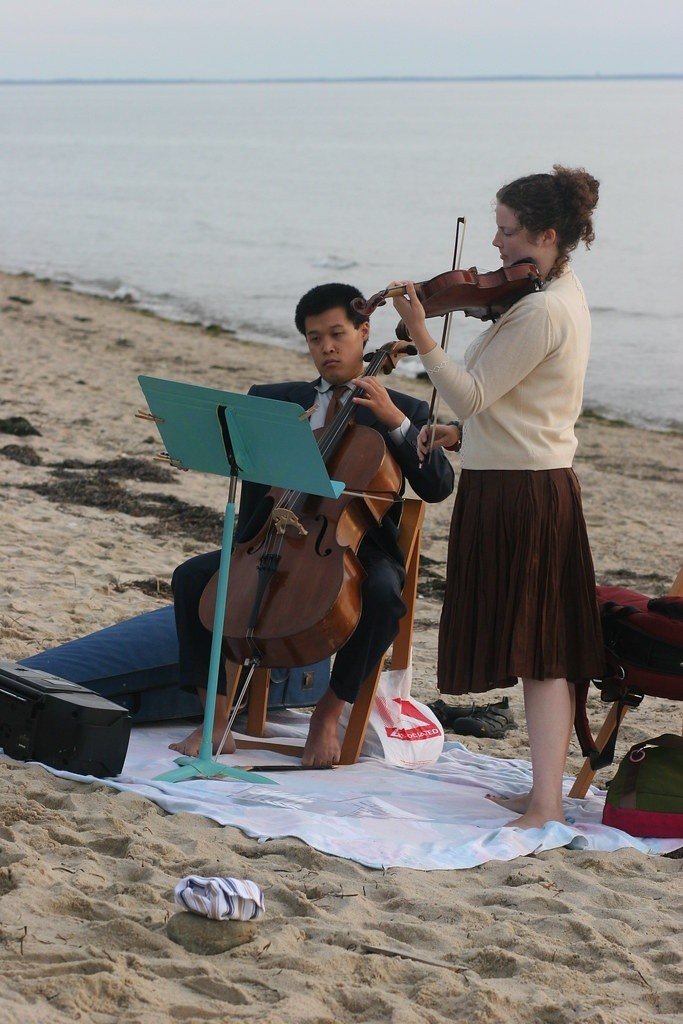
[444,421,462,452]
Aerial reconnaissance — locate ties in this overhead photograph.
[323,385,348,427]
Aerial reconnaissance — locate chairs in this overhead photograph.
[568,564,683,800]
[222,497,425,766]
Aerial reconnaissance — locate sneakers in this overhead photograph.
[426,700,487,729]
[456,696,519,739]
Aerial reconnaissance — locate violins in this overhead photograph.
[349,263,544,319]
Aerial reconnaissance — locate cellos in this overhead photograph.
[199,318,418,761]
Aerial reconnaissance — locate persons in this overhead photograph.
[392,166,608,829]
[167,284,454,769]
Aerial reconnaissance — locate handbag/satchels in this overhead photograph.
[591,585,683,707]
[601,735,683,840]
[336,668,445,766]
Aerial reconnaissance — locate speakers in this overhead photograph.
[0,661,132,778]
